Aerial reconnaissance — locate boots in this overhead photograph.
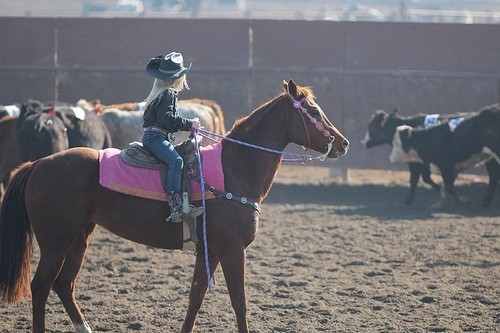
[167,192,204,223]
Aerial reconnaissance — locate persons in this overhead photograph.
[142,52,205,223]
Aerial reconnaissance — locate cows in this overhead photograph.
[388,102,499,213]
[0,99,224,201]
[361,109,470,206]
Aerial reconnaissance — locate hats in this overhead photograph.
[146,52,192,80]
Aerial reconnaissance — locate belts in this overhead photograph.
[143,126,173,142]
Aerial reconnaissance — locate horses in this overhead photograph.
[0,80,349,333]
